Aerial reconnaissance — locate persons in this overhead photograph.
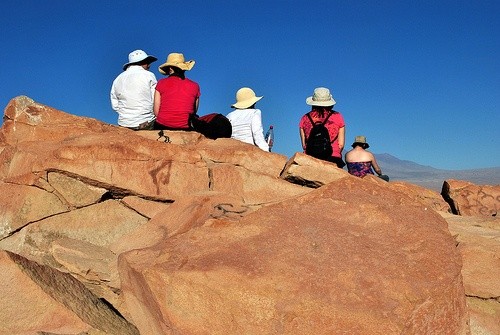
[110,50,159,131]
[345,136,389,182]
[153,52,200,131]
[300,87,346,168]
[226,87,270,152]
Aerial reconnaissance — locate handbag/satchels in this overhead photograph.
[188,113,232,139]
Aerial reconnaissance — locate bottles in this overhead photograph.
[265,126,275,152]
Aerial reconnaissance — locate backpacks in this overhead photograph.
[305,112,338,161]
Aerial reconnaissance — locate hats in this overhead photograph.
[158,53,195,74]
[306,87,336,106]
[123,50,158,70]
[230,88,264,109]
[351,136,370,149]
